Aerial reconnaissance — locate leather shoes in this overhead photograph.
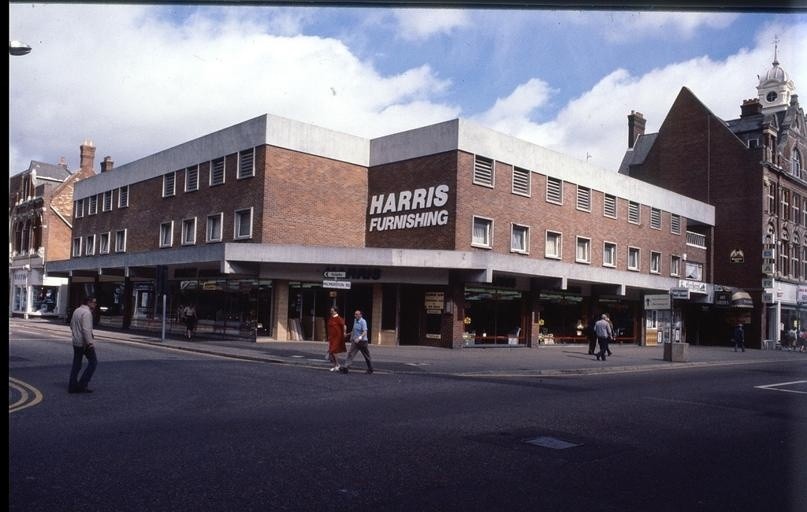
[597,354,599,360]
[366,368,374,375]
[339,367,348,374]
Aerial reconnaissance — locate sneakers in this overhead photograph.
[330,368,334,372]
[334,364,340,371]
[76,384,95,393]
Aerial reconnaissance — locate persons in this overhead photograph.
[326,306,347,372]
[594,314,613,361]
[181,301,199,340]
[733,323,747,352]
[782,324,807,351]
[338,309,374,373]
[588,313,598,354]
[67,292,99,394]
[604,314,616,357]
